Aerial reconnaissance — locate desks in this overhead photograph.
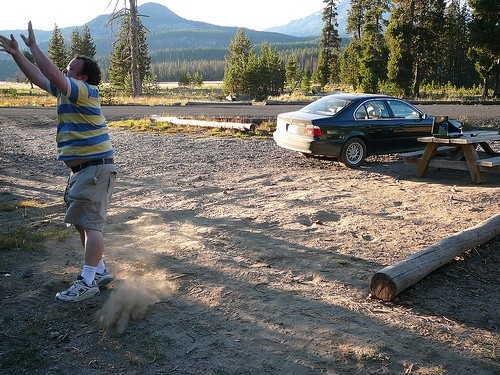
[415,131,500,184]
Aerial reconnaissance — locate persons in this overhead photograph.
[0,22,116,303]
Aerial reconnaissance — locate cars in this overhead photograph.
[275,92,462,169]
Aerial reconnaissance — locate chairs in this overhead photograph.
[364,106,387,119]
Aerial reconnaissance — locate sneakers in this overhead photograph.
[94,269,113,288]
[56,275,100,302]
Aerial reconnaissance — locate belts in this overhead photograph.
[70,158,114,174]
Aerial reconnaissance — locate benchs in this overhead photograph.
[398,146,457,161]
[474,156,500,168]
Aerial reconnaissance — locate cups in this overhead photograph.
[442,123,448,136]
